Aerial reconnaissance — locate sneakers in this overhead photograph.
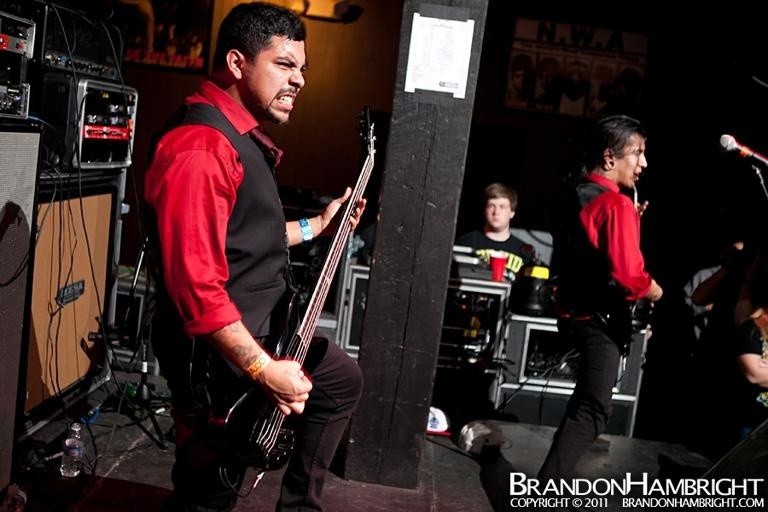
[590,437,613,450]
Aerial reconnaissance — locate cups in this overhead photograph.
[490,253,509,282]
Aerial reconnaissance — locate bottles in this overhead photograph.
[59,422,84,477]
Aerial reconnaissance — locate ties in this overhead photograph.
[247,127,286,173]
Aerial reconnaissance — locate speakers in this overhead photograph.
[17,185,118,425]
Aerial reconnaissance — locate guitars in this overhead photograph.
[586,197,650,357]
[204,107,378,472]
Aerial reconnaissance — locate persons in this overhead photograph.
[455,116,768,512]
[140,2,367,512]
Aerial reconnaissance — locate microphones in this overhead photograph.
[88,331,133,342]
[719,134,768,166]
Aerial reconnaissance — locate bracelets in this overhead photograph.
[244,351,272,378]
[297,217,313,242]
